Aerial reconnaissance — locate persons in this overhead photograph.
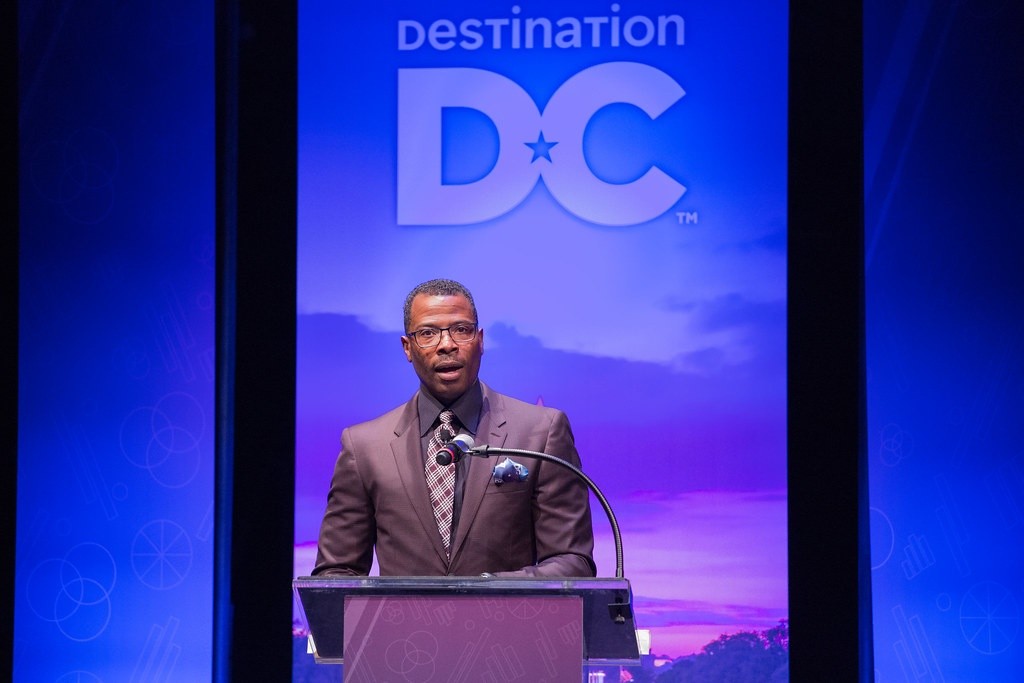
[311,279,597,578]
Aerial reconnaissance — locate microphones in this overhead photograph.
[435,434,474,466]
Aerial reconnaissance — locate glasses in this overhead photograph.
[407,322,478,348]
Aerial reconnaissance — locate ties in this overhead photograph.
[422,410,456,565]
